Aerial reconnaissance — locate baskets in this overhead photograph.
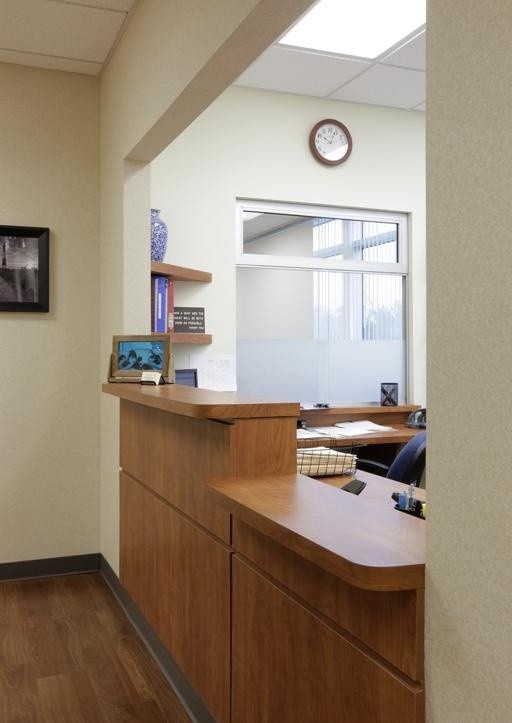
[296,433,367,477]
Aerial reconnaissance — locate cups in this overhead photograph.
[380,382,399,406]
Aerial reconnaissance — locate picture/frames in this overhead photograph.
[107,335,175,384]
[0,225,49,312]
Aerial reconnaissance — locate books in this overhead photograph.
[151,274,173,333]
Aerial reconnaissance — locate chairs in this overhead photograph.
[357,432,426,484]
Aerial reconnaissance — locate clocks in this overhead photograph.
[310,119,352,165]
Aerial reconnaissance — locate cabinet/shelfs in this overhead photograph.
[152,262,212,344]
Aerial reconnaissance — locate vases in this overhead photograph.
[151,209,168,263]
